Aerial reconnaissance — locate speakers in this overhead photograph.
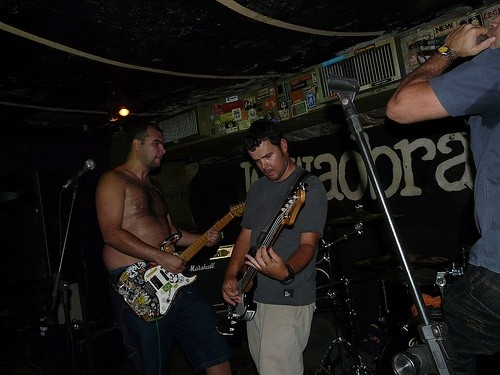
[186,240,237,311]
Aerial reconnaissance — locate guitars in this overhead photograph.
[220,183,308,323]
[114,202,248,324]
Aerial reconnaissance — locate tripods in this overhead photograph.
[314,224,371,375]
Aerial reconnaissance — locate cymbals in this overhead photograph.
[314,272,377,290]
[325,214,406,224]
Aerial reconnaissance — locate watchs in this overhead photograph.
[437,46,455,62]
[279,264,294,284]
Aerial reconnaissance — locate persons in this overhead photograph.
[386,14,500,375]
[95,123,232,375]
[223,119,327,375]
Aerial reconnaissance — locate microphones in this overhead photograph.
[62,159,96,190]
[422,33,489,47]
